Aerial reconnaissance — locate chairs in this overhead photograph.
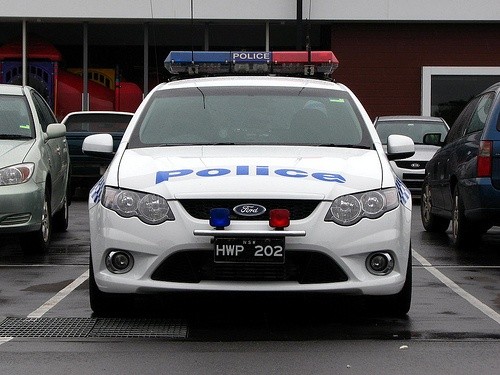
[290,109,327,129]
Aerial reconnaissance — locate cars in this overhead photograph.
[82,49,416,321]
[60,110,136,202]
[0,83,70,252]
[420,79,500,252]
[371,114,451,201]
